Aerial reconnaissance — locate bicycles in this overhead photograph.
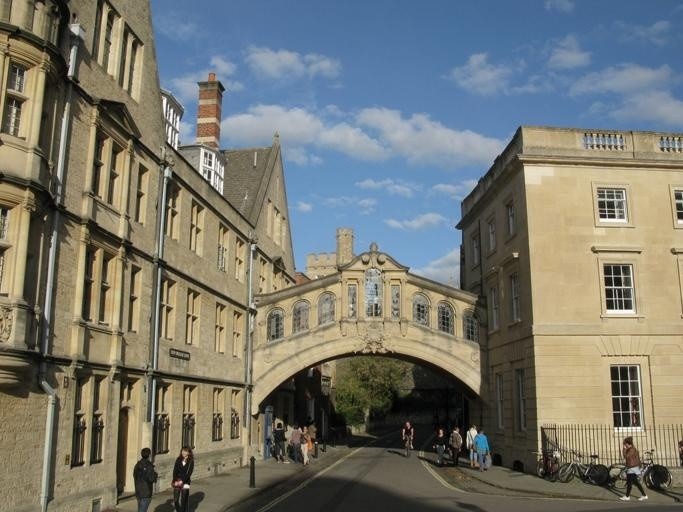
[403,435,413,457]
[536,447,673,490]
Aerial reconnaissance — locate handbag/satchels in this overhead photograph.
[484,455,492,469]
[171,479,184,489]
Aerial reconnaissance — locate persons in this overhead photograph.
[272,422,292,464]
[286,414,317,467]
[169,445,194,512]
[432,427,447,466]
[132,447,161,512]
[466,424,478,467]
[677,441,682,468]
[471,428,490,472]
[448,425,461,466]
[447,428,462,457]
[618,436,648,501]
[401,420,414,450]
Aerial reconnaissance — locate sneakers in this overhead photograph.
[638,494,648,501]
[619,495,630,501]
